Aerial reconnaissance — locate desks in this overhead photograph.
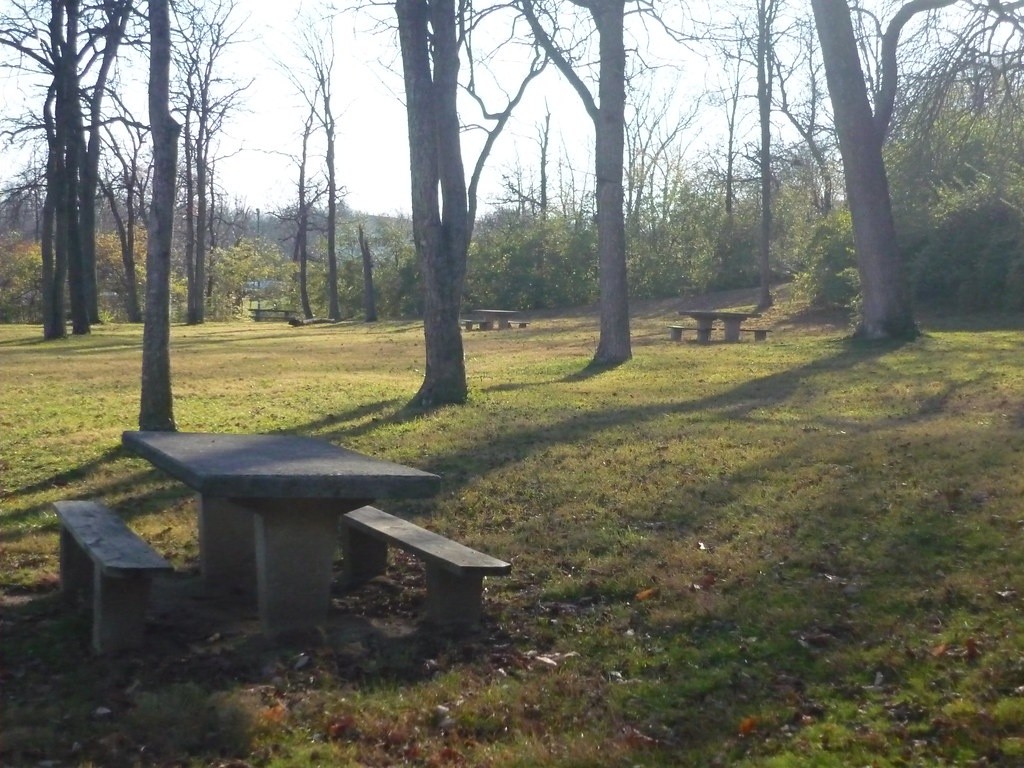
[679,311,762,342]
[122,430,442,645]
[472,310,519,330]
[248,309,297,322]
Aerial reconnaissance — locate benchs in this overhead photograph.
[464,319,490,330]
[508,321,530,329]
[340,505,512,633]
[720,327,772,341]
[51,500,174,655]
[249,315,284,319]
[667,325,716,343]
